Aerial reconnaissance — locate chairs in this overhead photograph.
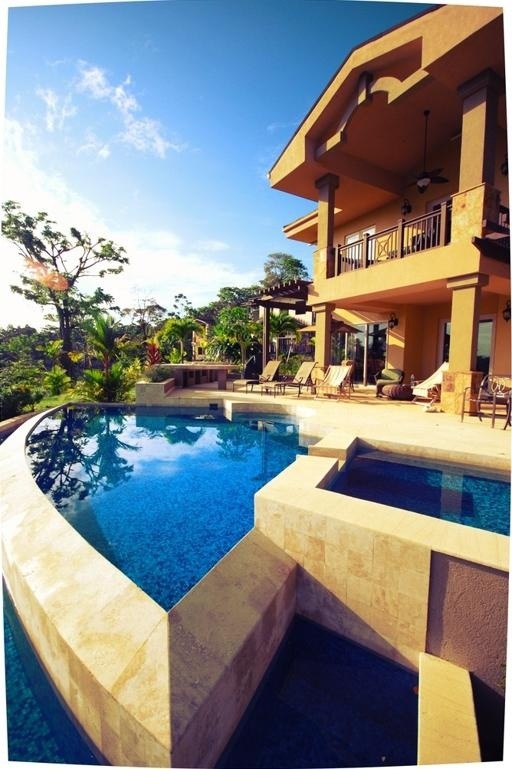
[410,360,450,407]
[460,375,512,428]
[373,368,405,397]
[232,359,355,401]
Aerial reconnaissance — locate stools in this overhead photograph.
[382,383,414,400]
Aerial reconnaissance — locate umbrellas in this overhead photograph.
[296,323,362,334]
[369,328,387,336]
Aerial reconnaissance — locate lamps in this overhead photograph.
[502,299,511,322]
[388,313,399,329]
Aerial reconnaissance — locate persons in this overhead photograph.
[352,338,364,383]
